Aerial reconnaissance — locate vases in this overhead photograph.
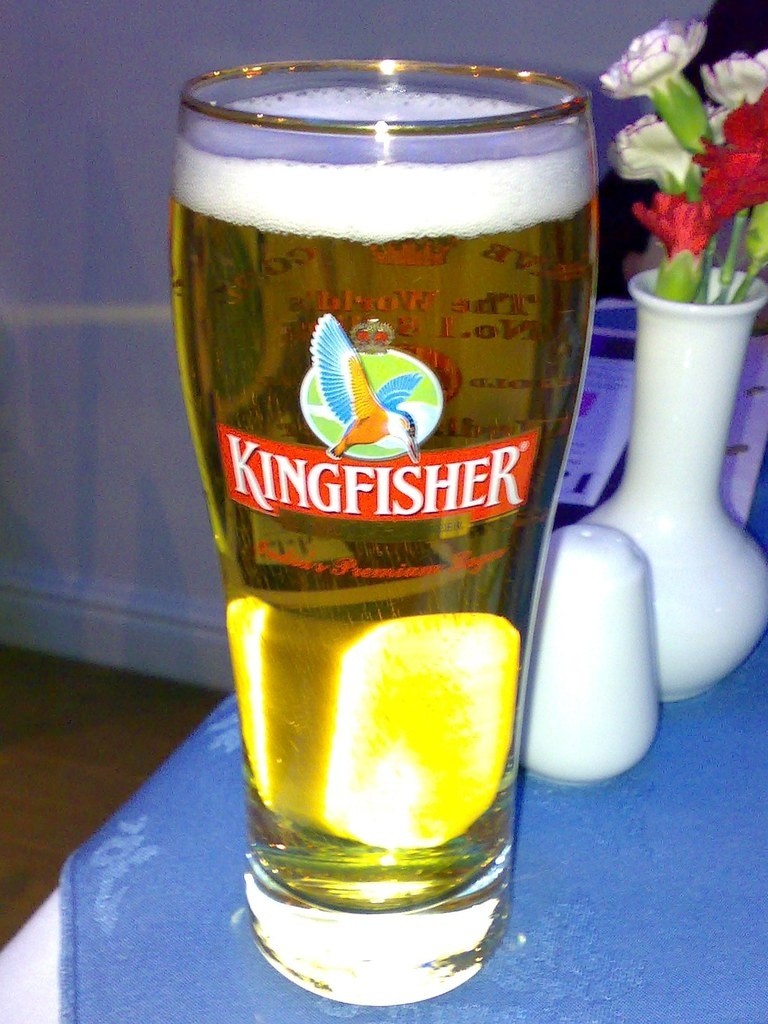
[577,268,768,702]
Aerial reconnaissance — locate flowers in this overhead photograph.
[598,16,768,303]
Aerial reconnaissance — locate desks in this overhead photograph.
[0,503,768,1024]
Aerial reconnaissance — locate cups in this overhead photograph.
[170,60,598,1005]
